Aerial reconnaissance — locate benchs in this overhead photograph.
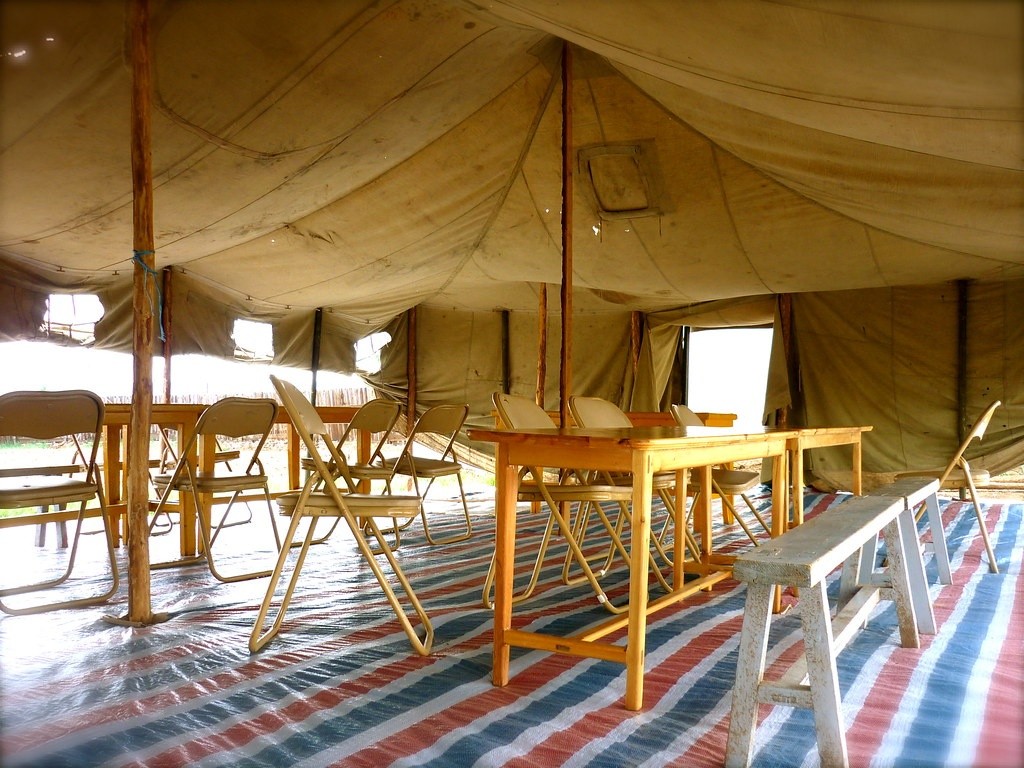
[724,494,923,768]
[858,477,953,635]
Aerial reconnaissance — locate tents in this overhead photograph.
[0,0,1022,628]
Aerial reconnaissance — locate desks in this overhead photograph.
[526,409,739,533]
[121,404,371,556]
[709,426,872,599]
[466,423,804,710]
[100,402,205,557]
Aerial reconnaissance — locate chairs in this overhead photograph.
[1,374,1001,657]
